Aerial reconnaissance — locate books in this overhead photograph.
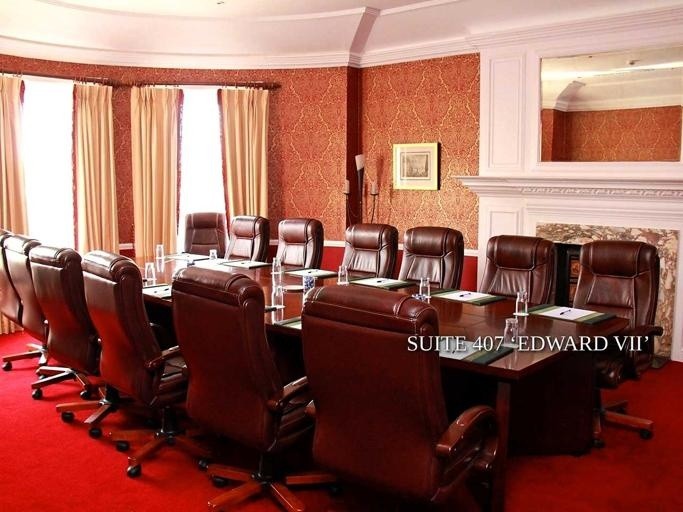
[435,336,515,366]
[217,259,271,269]
[283,268,338,279]
[275,309,302,332]
[166,252,209,263]
[347,276,416,291]
[519,303,616,326]
[428,284,508,306]
[139,283,178,299]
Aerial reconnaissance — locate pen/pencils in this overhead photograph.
[154,288,169,293]
[376,280,386,283]
[460,292,472,297]
[307,271,316,274]
[559,309,572,315]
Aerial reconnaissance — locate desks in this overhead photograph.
[118,250,630,473]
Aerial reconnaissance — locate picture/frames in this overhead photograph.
[391,142,439,191]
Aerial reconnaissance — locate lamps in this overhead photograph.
[343,152,378,224]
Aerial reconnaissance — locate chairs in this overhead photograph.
[180,210,663,451]
[1,229,55,380]
[29,247,168,437]
[1,236,94,400]
[83,251,212,478]
[170,267,345,512]
[300,285,501,512]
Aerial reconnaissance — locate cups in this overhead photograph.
[270,287,283,307]
[144,280,155,287]
[143,263,154,280]
[208,249,217,264]
[515,314,527,335]
[272,273,283,288]
[270,307,283,324]
[502,347,518,369]
[501,319,519,346]
[155,244,164,260]
[514,291,528,313]
[270,256,281,274]
[419,277,430,297]
[155,258,164,273]
[336,265,349,284]
[420,298,429,305]
